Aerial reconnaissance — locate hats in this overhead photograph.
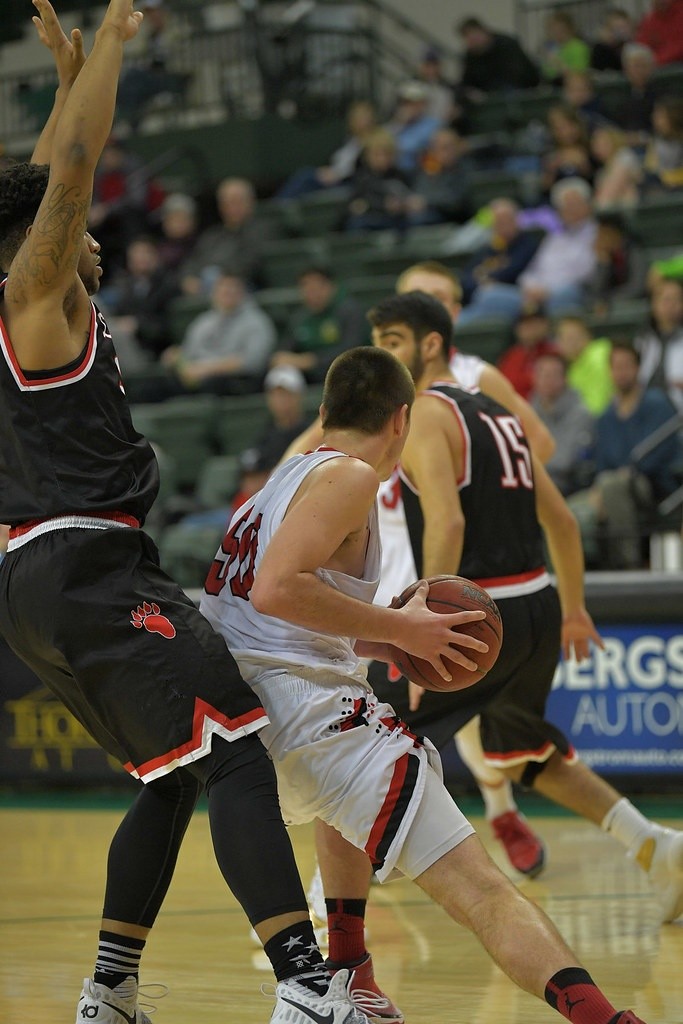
[267,364,304,392]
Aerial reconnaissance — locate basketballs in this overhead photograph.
[390,574,503,690]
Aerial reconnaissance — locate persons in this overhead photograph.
[0,1,377,1024]
[201,346,653,1024]
[0,0,683,922]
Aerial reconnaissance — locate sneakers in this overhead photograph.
[615,1010,646,1024]
[259,968,390,1023]
[490,809,545,877]
[628,826,682,922]
[325,951,406,1023]
[73,978,168,1024]
[250,897,329,946]
